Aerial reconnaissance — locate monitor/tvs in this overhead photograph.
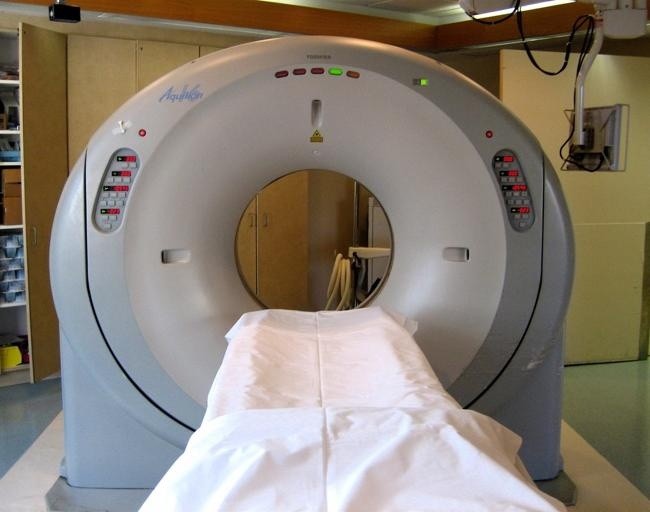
[567,105,629,171]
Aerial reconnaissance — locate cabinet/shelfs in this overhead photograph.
[0,0,309,389]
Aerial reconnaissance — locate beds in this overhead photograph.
[138,305,567,512]
